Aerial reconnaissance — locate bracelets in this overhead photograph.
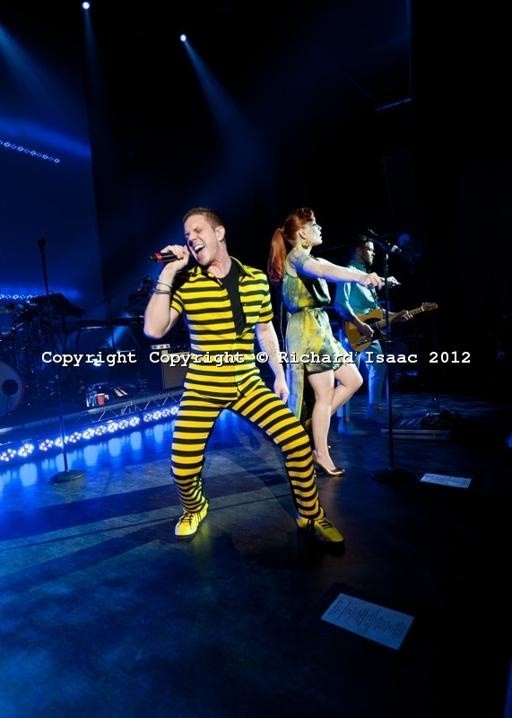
[153,281,173,295]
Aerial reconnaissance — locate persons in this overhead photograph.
[333,234,415,437]
[128,276,153,338]
[265,207,403,478]
[142,205,347,547]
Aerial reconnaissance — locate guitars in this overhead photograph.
[346,302,438,352]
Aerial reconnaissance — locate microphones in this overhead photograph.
[39,239,46,263]
[149,251,178,262]
[358,227,400,255]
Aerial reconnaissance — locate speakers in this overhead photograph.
[148,349,190,390]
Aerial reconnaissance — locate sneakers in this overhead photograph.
[337,407,384,437]
[174,500,211,541]
[294,510,345,546]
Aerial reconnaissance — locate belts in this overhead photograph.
[289,303,324,314]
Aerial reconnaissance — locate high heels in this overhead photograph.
[311,450,347,478]
